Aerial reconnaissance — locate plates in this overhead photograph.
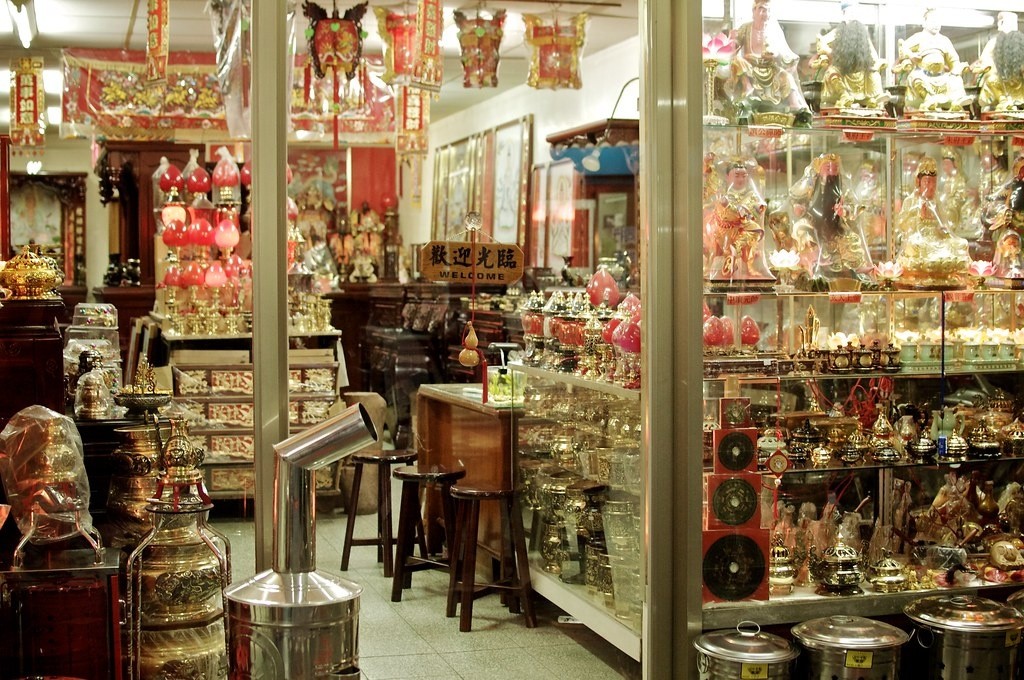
[880,367,902,373]
[852,368,875,374]
[830,369,852,374]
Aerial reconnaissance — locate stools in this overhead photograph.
[447,482,520,633]
[341,449,428,578]
[392,465,465,603]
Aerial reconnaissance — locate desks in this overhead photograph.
[0,548,126,680]
[417,382,525,588]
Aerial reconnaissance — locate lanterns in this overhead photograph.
[453,7,506,90]
[522,7,583,92]
[374,0,443,208]
[302,1,370,156]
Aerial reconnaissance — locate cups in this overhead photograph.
[796,359,816,375]
[831,334,1024,368]
[819,358,828,372]
[777,360,794,374]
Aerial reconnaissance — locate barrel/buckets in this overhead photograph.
[791,615,915,680]
[521,461,641,636]
[693,619,802,680]
[902,594,1024,680]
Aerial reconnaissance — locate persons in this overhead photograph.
[856,143,1024,281]
[898,9,973,110]
[979,13,1024,112]
[710,159,765,278]
[789,153,874,273]
[807,33,986,84]
[815,0,892,106]
[730,0,807,112]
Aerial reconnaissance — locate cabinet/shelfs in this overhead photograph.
[157,326,342,499]
[0,292,73,430]
[91,138,208,370]
[508,284,1024,680]
[321,282,508,449]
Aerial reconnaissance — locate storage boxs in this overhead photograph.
[703,122,1024,285]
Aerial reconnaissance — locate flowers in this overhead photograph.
[703,31,742,124]
